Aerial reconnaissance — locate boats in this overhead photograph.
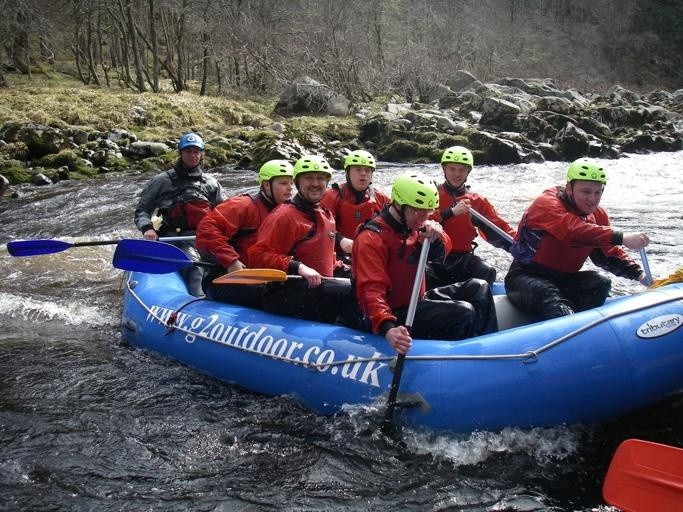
[116,232,682,436]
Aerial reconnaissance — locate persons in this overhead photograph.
[321,150,390,256]
[348,171,498,355]
[246,155,352,329]
[503,155,650,320]
[196,159,294,313]
[430,146,517,290]
[133,133,228,297]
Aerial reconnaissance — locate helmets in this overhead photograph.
[392,145,474,210]
[259,150,376,191]
[567,157,606,183]
[179,133,205,150]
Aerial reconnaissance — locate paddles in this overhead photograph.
[212,269,352,286]
[7,235,199,257]
[111,239,225,274]
